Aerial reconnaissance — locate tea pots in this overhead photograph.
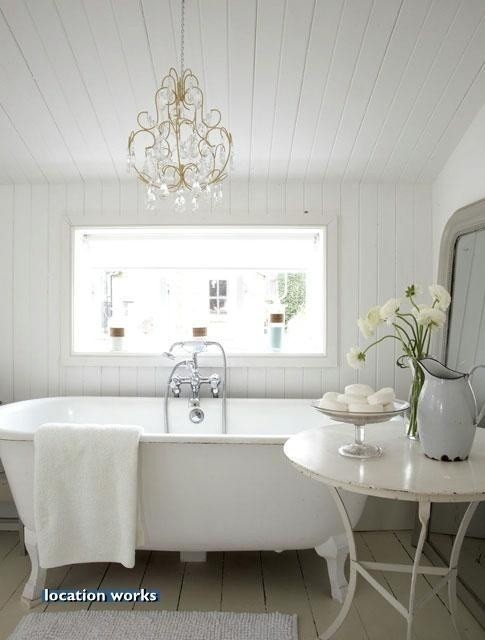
[417,357,485,462]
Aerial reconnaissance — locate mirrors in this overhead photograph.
[432,199,485,429]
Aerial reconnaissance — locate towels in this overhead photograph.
[33,424,139,568]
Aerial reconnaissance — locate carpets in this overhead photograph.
[6,609,298,640]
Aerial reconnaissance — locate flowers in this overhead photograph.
[346,284,451,436]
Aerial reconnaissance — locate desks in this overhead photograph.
[284,419,485,640]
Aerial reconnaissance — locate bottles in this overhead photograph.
[109,326,124,352]
[269,311,286,355]
[190,327,208,352]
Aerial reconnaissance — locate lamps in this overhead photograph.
[124,0,235,213]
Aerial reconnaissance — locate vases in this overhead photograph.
[406,352,435,440]
